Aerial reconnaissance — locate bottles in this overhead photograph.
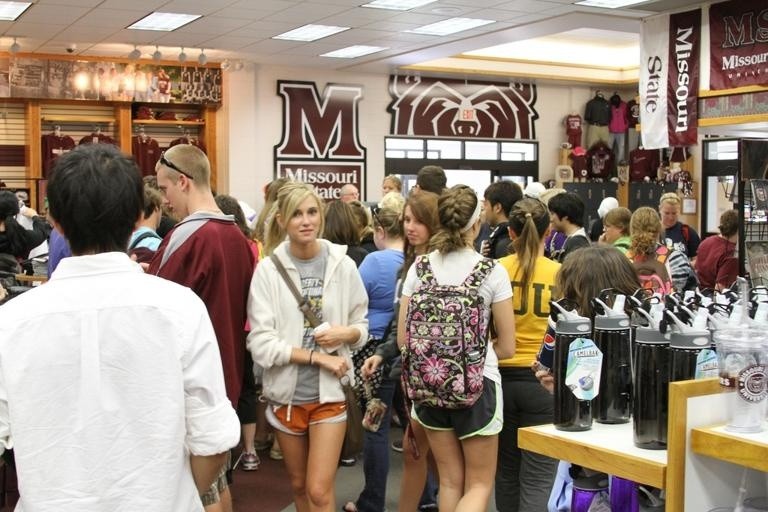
[567,465,642,511]
[551,282,763,454]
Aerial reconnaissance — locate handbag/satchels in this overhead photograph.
[343,382,364,445]
[0,253,24,305]
[362,398,388,433]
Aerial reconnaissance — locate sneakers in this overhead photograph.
[238,432,283,470]
[338,457,358,466]
[392,439,404,451]
[343,502,358,511]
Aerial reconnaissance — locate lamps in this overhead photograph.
[0,34,244,72]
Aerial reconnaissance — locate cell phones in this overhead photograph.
[314,322,343,355]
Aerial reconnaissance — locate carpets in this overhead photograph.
[229,451,294,512]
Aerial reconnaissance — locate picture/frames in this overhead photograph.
[681,197,697,215]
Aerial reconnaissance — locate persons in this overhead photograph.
[241,166,653,512]
[658,192,703,277]
[626,205,699,296]
[1,141,258,512]
[690,209,740,297]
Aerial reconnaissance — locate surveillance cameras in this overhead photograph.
[66,42,77,54]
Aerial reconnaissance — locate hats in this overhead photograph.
[524,181,567,206]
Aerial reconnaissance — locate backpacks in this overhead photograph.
[401,255,499,410]
[627,247,674,301]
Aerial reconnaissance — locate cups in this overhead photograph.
[714,329,767,435]
[533,313,555,377]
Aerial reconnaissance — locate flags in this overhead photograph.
[637,7,704,151]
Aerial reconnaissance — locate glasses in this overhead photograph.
[373,208,386,230]
[159,150,192,180]
